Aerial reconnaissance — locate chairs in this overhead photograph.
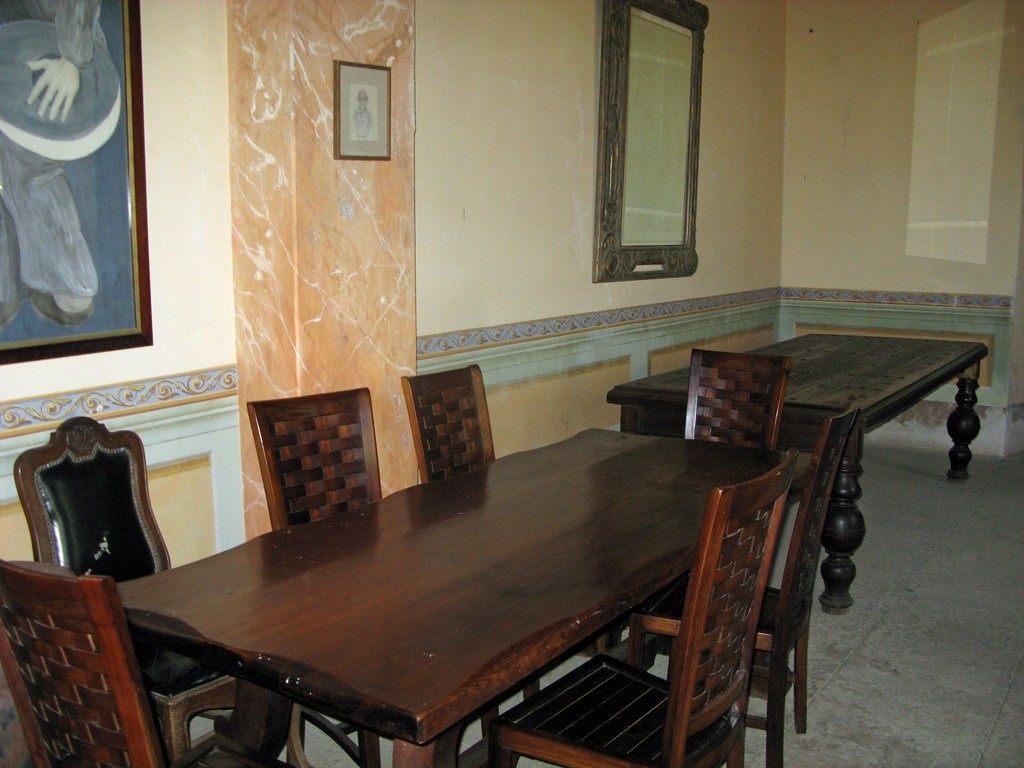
[624,403,858,768]
[625,347,793,675]
[245,386,382,768]
[401,364,606,699]
[13,417,236,766]
[0,555,294,768]
[488,450,801,768]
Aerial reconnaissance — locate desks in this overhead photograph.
[115,426,818,768]
[606,333,987,616]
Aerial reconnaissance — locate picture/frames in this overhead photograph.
[332,60,392,160]
[592,0,709,282]
[0,0,153,366]
